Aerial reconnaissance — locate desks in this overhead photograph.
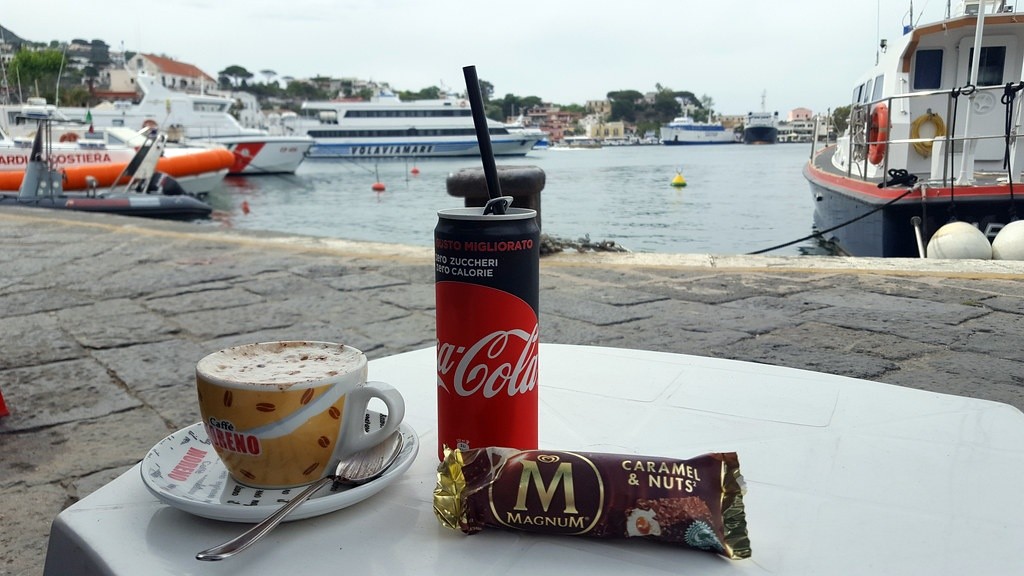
[43,342,1024,576]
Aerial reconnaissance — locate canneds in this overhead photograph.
[434,196,541,464]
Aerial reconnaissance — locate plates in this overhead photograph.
[140,408,420,524]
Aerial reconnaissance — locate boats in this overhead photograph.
[656,96,745,145]
[776,114,816,144]
[0,110,235,196]
[272,92,552,158]
[803,0,1024,257]
[741,89,779,146]
[0,42,320,202]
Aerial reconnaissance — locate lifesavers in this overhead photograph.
[866,104,888,164]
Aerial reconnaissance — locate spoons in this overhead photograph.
[193,429,403,563]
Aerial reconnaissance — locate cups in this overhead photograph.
[193,339,406,490]
